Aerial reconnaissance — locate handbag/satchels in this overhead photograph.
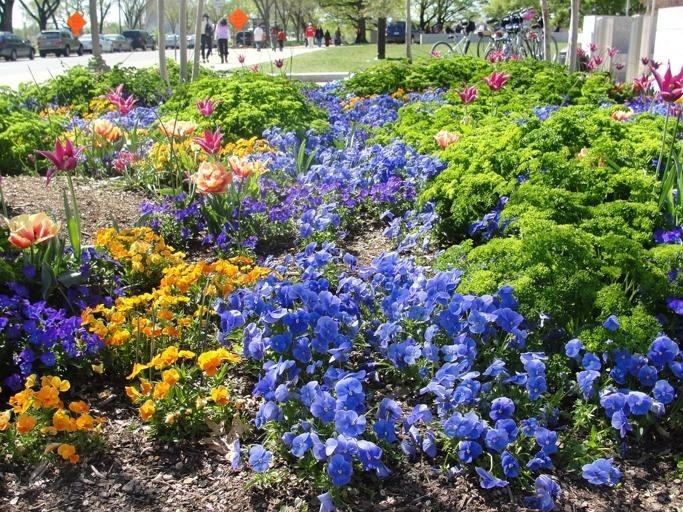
[205,23,211,35]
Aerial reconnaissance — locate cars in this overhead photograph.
[78,30,256,55]
[1,30,37,62]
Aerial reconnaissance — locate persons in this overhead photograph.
[213,16,231,64]
[304,22,341,49]
[269,27,276,52]
[253,24,263,53]
[198,12,213,64]
[276,29,287,52]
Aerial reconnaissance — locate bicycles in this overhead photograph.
[429,6,560,63]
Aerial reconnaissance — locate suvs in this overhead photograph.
[385,20,428,44]
[37,28,86,61]
